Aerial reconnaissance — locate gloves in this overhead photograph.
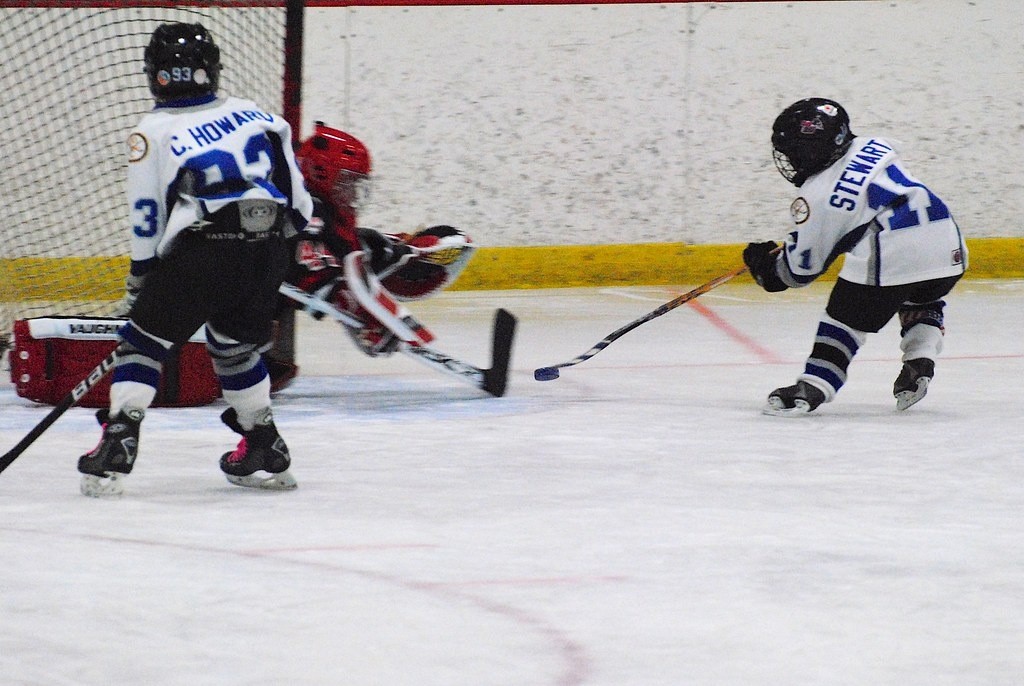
[331,286,402,359]
[125,274,147,312]
[743,240,790,292]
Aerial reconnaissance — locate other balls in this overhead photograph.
[535,366,559,380]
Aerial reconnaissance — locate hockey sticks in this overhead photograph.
[276,280,517,398]
[535,242,788,382]
[0,343,123,474]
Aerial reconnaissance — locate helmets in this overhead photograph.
[295,125,371,193]
[770,97,858,187]
[144,21,221,96]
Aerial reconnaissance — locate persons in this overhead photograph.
[77,22,314,496]
[270,121,476,398]
[742,98,972,413]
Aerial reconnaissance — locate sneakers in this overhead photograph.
[892,358,935,410]
[219,407,299,490]
[767,380,825,416]
[76,407,145,498]
